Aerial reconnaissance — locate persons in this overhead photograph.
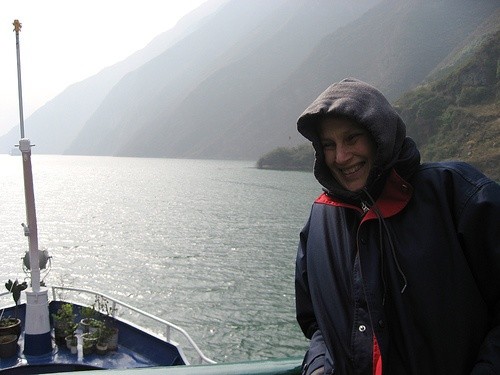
[296,78,500,375]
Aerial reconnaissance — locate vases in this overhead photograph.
[0,335,18,358]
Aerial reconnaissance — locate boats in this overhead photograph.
[0,19,217,374]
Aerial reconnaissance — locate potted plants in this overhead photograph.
[0,280,28,341]
[52,295,119,355]
